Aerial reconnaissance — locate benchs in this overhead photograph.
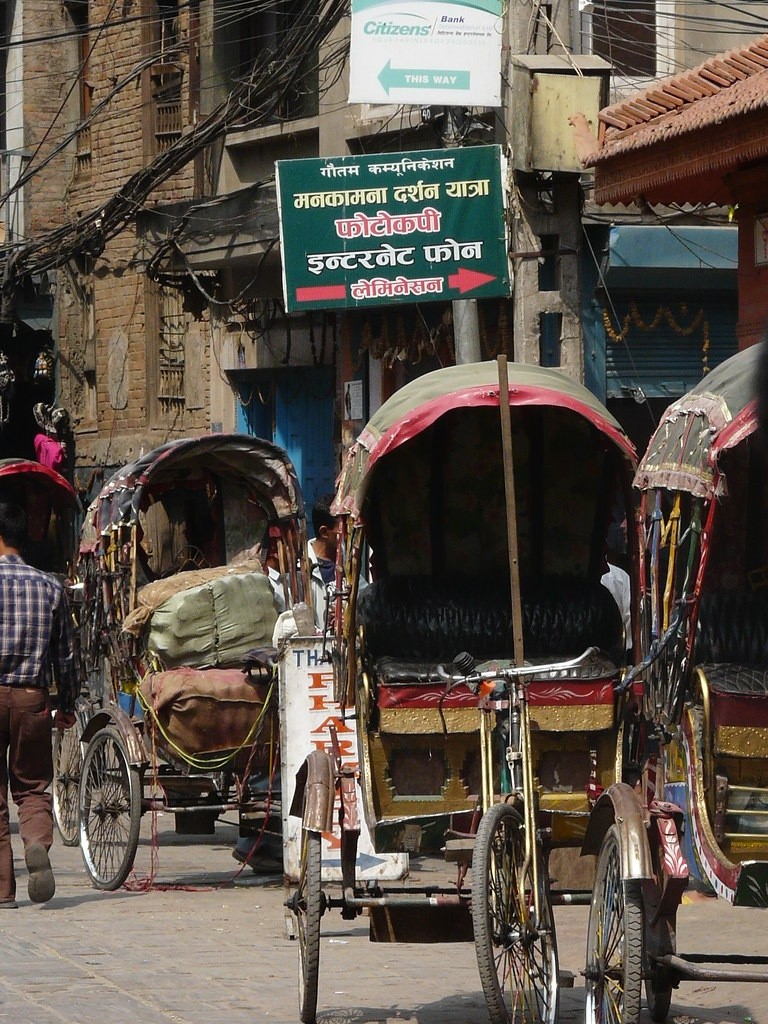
[353,567,622,737]
[673,588,768,761]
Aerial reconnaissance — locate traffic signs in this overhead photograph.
[276,144,512,313]
[347,1,502,107]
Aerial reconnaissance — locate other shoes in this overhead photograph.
[0,900,18,909]
[232,844,283,873]
[24,843,55,904]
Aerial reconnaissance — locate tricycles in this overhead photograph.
[288,357,644,1022]
[579,335,768,1023]
[77,430,316,890]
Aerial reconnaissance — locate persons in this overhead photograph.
[232,518,305,874]
[306,493,369,630]
[0,502,83,909]
